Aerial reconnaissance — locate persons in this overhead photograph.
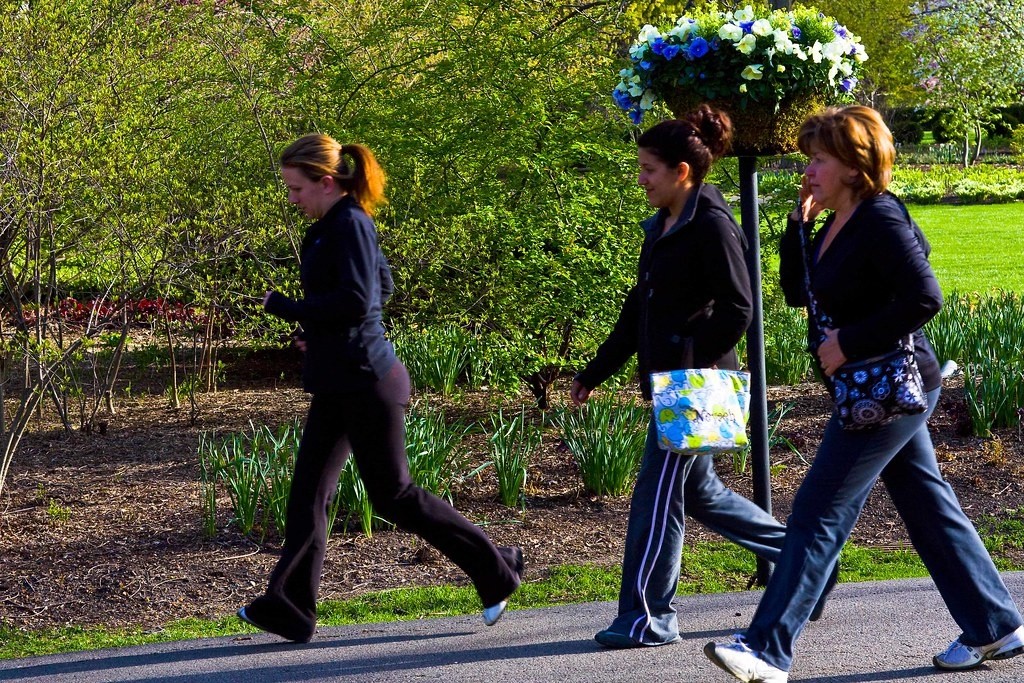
[705,107,1024,683]
[236,134,523,644]
[569,103,840,648]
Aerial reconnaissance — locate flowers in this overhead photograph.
[610,4,870,125]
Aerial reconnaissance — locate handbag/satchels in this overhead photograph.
[820,346,927,437]
[649,368,751,455]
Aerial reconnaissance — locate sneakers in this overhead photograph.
[703,633,788,683]
[932,622,1024,672]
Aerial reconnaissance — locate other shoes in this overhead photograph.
[808,558,838,621]
[595,629,681,649]
[236,607,267,631]
[481,596,511,626]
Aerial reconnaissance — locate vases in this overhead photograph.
[659,92,825,158]
